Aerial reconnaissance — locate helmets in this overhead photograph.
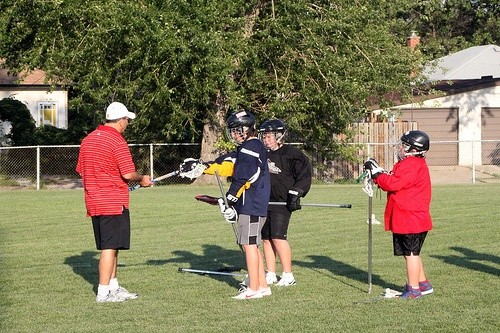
[398,130,430,160]
[257,119,286,152]
[226,110,256,147]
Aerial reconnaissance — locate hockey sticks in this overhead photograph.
[178,267,249,277]
[215,170,239,244]
[195,194,351,209]
[363,169,373,294]
[130,157,208,191]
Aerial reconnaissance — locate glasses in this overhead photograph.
[123,117,130,123]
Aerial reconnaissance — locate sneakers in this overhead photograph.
[231,285,264,299]
[396,285,421,299]
[400,283,434,295]
[276,275,296,286]
[111,285,138,298]
[258,287,272,296]
[96,290,125,302]
[265,272,278,284]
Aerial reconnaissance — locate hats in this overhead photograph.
[105,101,135,121]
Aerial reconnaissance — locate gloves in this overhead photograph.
[287,190,299,211]
[221,193,240,224]
[181,160,209,173]
[364,158,385,179]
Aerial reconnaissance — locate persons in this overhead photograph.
[364,130,434,299]
[76,102,155,302]
[217,111,272,299]
[180,120,312,286]
